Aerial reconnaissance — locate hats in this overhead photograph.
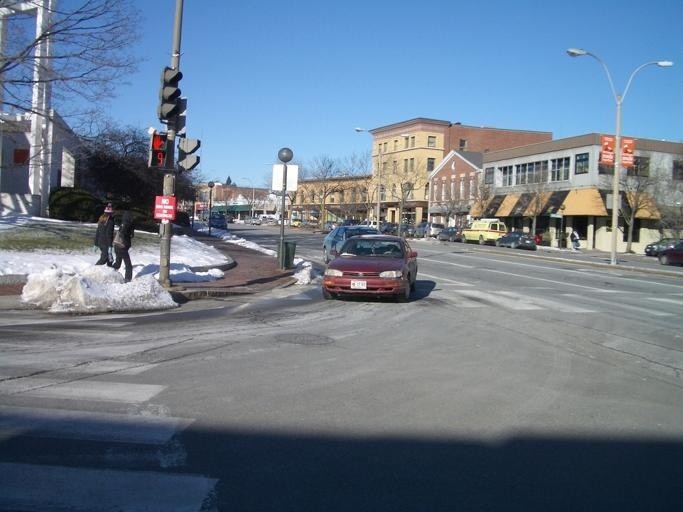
[122,211,132,225]
[104,202,115,213]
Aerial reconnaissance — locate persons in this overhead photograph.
[570,227,579,251]
[94,202,114,266]
[113,206,136,283]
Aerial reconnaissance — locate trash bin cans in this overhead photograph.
[276,240,296,267]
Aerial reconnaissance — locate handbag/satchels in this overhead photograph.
[113,230,128,248]
[571,233,580,246]
